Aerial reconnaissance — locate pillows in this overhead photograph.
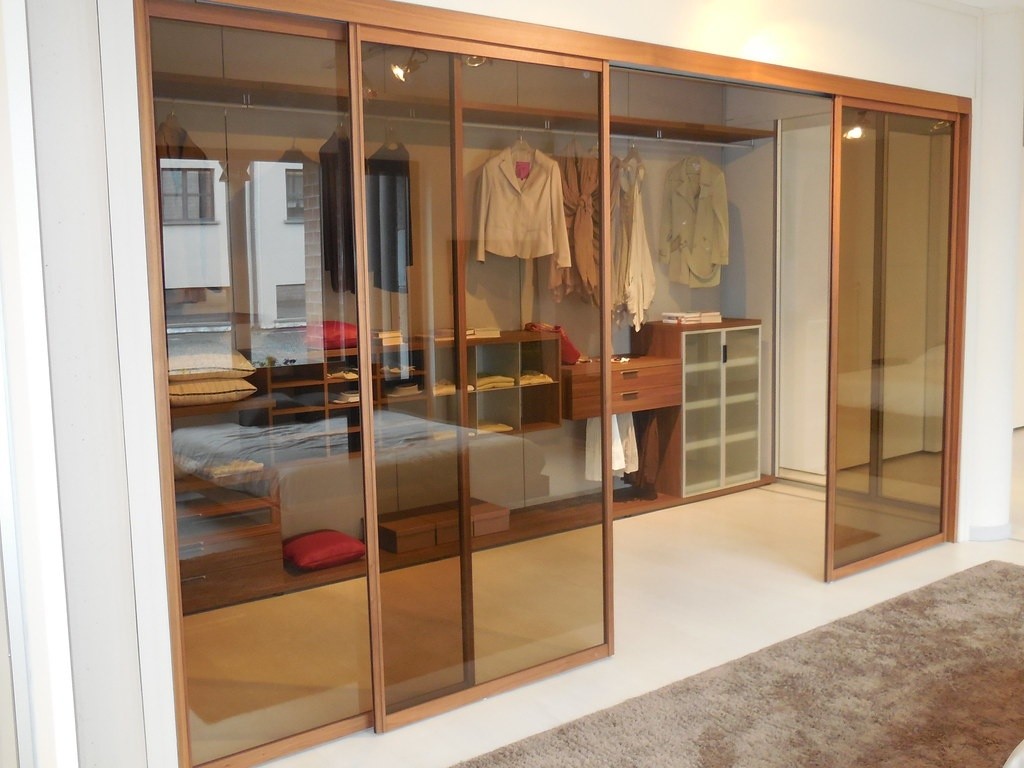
[166,339,257,381]
[266,321,357,351]
[169,378,258,407]
[282,529,366,573]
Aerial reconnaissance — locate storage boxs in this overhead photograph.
[361,496,511,555]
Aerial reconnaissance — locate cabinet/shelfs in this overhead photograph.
[239,329,560,469]
[630,318,762,499]
[174,465,284,616]
[560,353,682,422]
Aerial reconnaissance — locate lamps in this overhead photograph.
[463,55,492,68]
[391,49,429,82]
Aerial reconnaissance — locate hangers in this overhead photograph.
[158,101,722,181]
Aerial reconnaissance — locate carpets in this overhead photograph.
[448,560,1024,768]
